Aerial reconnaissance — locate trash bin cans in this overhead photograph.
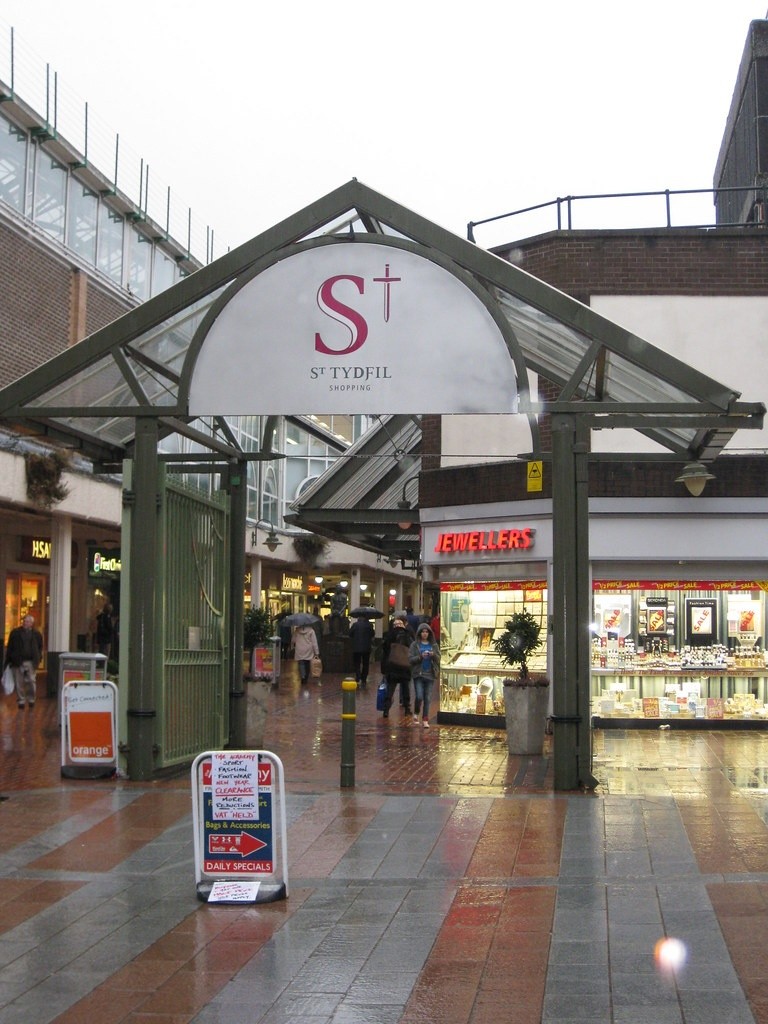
[57,652,110,727]
[252,635,281,687]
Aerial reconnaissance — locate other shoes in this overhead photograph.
[301,676,308,683]
[383,712,388,717]
[423,718,430,728]
[405,709,412,716]
[356,676,367,683]
[413,714,419,724]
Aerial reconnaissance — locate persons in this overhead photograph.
[390,607,440,647]
[329,585,347,635]
[461,603,472,622]
[289,626,319,685]
[407,623,440,728]
[96,604,113,668]
[276,615,291,660]
[381,620,414,717]
[349,615,375,688]
[4,616,42,708]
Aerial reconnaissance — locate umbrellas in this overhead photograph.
[284,612,321,625]
[348,606,384,619]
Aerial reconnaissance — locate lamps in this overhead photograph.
[252,518,282,552]
[397,476,419,529]
[389,590,397,595]
[339,581,348,588]
[315,576,323,583]
[676,458,715,497]
[360,585,367,590]
[377,553,402,568]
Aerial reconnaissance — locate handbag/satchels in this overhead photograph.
[376,683,389,710]
[389,643,412,668]
[311,656,323,678]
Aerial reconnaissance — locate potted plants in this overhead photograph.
[493,607,549,755]
